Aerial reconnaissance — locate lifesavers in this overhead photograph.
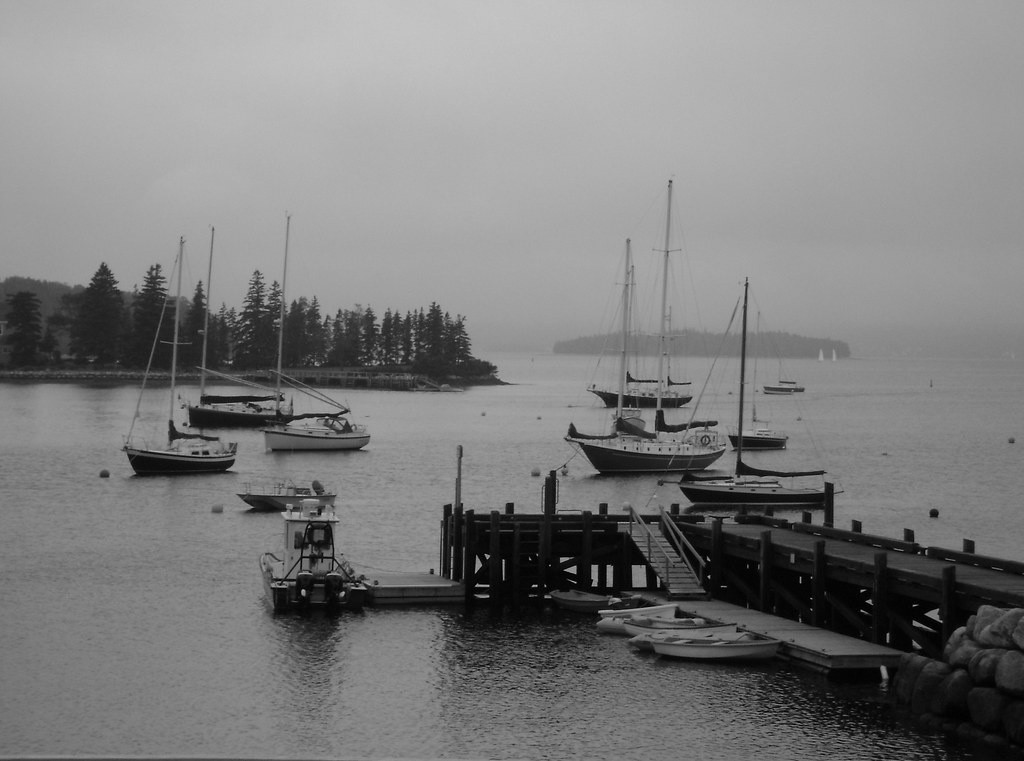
[701,434,711,446]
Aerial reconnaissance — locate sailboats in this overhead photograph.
[653,275,846,508]
[583,236,809,453]
[256,210,371,454]
[176,225,296,429]
[119,235,239,476]
[562,178,729,475]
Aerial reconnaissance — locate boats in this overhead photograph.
[259,497,368,615]
[549,588,785,662]
[234,477,340,514]
